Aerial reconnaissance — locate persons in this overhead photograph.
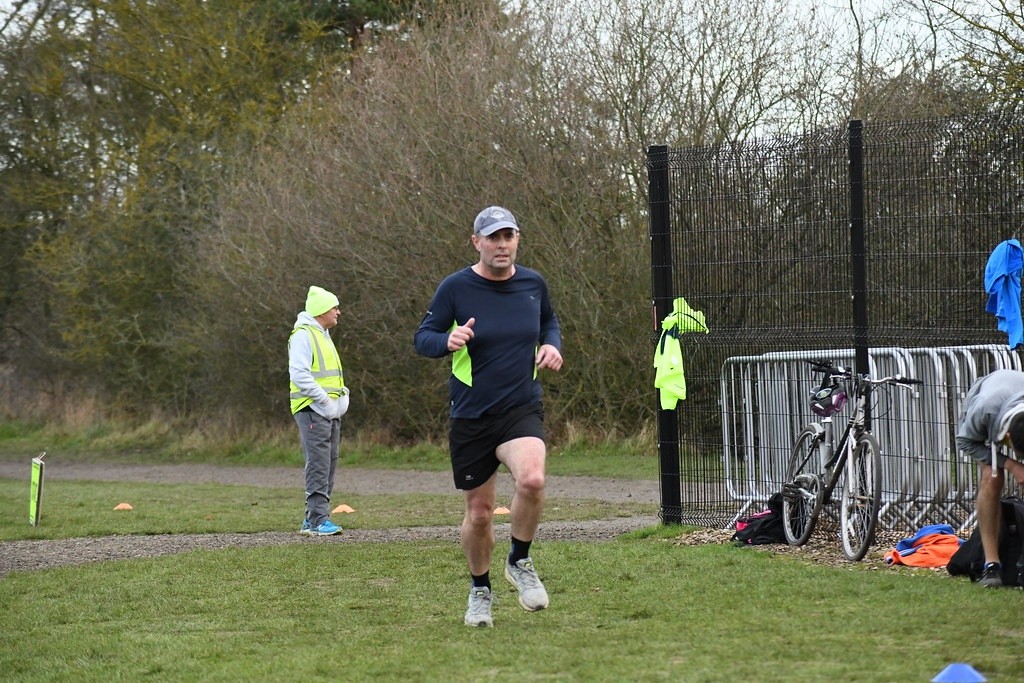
[286,286,350,535]
[413,205,563,630]
[955,368,1024,587]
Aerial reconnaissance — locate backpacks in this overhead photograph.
[947,495,1024,585]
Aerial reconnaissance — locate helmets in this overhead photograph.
[810,384,847,418]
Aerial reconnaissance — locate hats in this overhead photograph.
[473,205,520,237]
[304,286,340,319]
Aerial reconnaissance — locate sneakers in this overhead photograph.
[504,551,550,612]
[981,561,1003,588]
[463,585,494,627]
[300,519,343,535]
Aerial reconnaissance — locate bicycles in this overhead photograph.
[780,356,927,561]
